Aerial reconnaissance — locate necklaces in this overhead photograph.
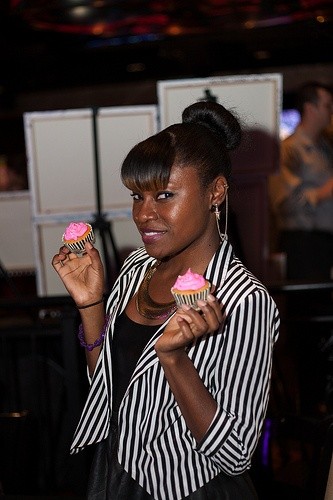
[133,273,179,320]
[143,260,176,310]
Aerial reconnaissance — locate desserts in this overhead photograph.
[60,221,97,254]
[170,268,212,311]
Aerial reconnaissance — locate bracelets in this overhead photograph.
[76,312,108,352]
[48,100,280,497]
[74,299,103,311]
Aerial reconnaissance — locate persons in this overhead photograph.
[266,78,332,318]
